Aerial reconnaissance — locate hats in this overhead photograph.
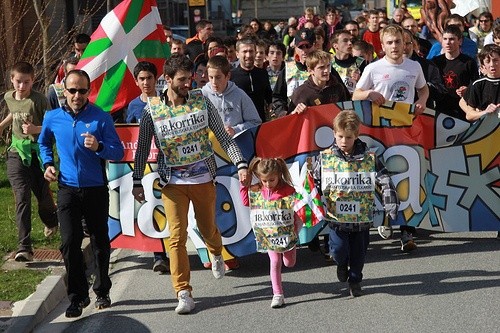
[295,28,315,46]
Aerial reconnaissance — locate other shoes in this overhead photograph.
[225,258,240,270]
[153,260,168,272]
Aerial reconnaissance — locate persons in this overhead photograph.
[155,2,500,121]
[37,70,124,318]
[50,33,125,236]
[126,60,170,274]
[286,50,347,260]
[201,55,262,269]
[311,110,399,297]
[131,52,252,312]
[352,25,429,252]
[240,156,303,307]
[459,44,500,239]
[0,61,58,261]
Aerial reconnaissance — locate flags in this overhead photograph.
[290,162,328,228]
[75,0,172,114]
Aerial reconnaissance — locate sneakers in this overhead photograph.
[65,298,90,317]
[15,252,33,261]
[350,284,361,296]
[175,290,194,313]
[44,225,57,240]
[378,226,393,240]
[337,264,348,282]
[95,294,111,308]
[210,254,225,278]
[271,295,285,307]
[401,233,417,251]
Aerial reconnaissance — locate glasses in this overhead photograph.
[298,45,312,49]
[197,71,208,77]
[479,19,490,23]
[66,88,88,94]
